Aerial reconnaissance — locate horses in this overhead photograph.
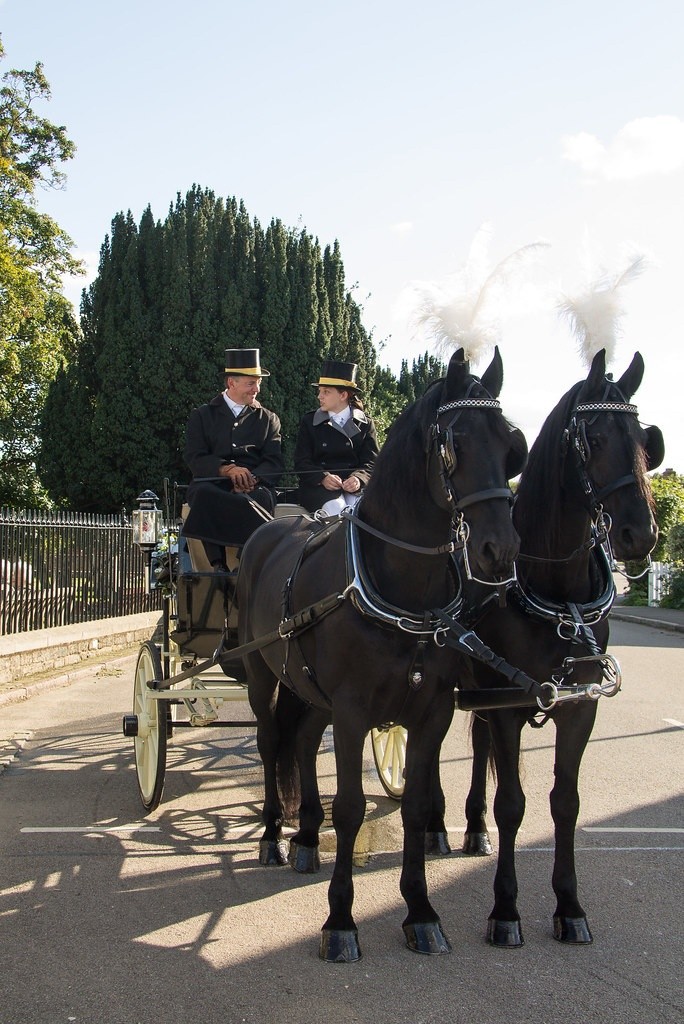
[236,342,526,962]
[436,349,664,949]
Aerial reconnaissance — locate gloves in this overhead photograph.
[224,463,257,493]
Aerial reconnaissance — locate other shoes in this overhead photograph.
[213,561,230,589]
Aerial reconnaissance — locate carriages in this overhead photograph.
[122,345,662,964]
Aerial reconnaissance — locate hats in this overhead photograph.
[310,360,362,392]
[217,349,270,377]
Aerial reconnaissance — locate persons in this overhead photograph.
[10,561,33,593]
[182,349,281,574]
[0,558,17,609]
[296,359,381,517]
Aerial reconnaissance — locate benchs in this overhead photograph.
[177,496,309,574]
